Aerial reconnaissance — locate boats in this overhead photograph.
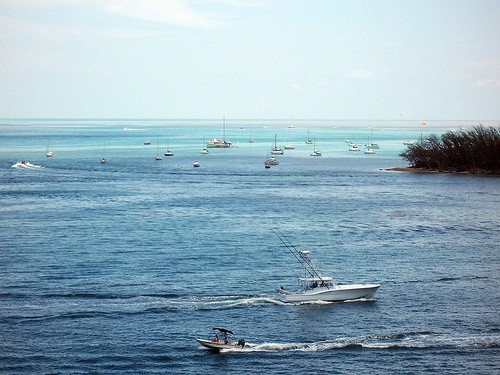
[284,144,295,149]
[47,152,53,156]
[196,338,251,349]
[101,159,105,163]
[192,160,200,167]
[207,139,231,148]
[263,157,279,165]
[144,140,150,145]
[276,275,381,303]
[289,126,294,128]
[344,128,430,154]
[249,138,253,143]
[156,158,162,160]
[200,148,208,154]
[165,151,174,156]
[21,160,26,164]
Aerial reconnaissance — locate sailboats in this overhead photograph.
[310,137,321,156]
[270,133,284,154]
[265,150,271,168]
[305,129,312,144]
